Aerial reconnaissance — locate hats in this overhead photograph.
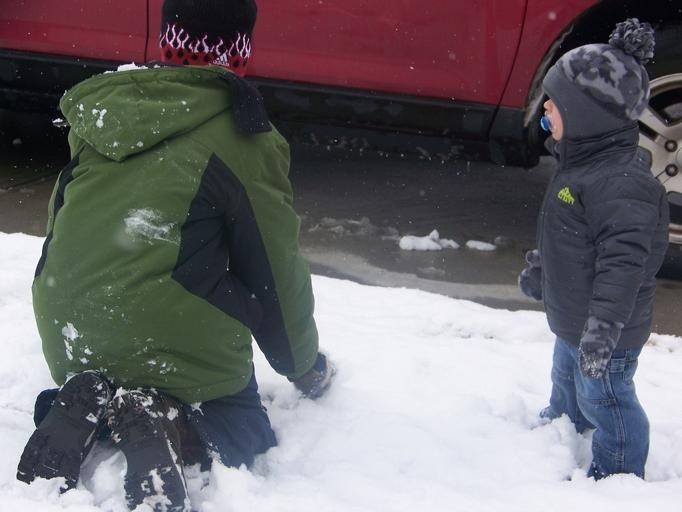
[541,18,656,140]
[160,1,256,77]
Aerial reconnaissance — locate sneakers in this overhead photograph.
[108,387,191,511]
[15,372,111,495]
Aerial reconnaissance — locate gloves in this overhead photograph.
[292,353,336,402]
[579,314,625,381]
[516,247,546,306]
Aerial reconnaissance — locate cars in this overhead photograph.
[2,3,682,283]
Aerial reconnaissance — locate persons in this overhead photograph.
[515,17,670,477]
[15,0,336,511]
